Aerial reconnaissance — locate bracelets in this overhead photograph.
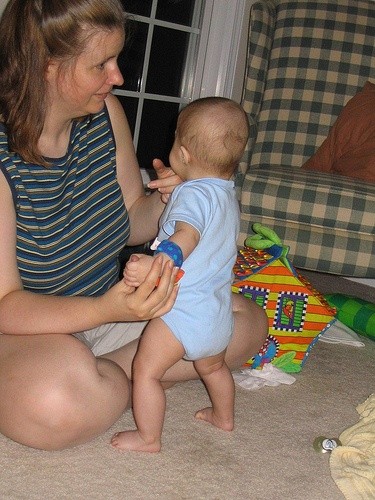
[153,239,184,270]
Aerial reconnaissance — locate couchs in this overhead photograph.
[234,0,374,279]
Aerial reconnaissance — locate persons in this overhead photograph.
[110,97,249,451]
[0,0,268,452]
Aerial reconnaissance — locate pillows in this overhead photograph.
[301,80,375,182]
